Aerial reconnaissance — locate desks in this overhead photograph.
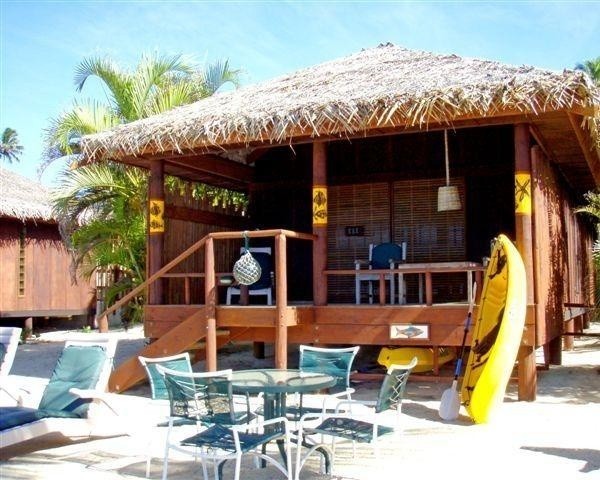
[211,369,338,479]
[398,261,430,304]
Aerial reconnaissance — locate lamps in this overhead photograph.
[437,130,462,211]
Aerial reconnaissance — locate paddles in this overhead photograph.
[439,281,477,420]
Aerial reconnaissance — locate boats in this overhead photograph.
[377,346,456,373]
[461,234,527,424]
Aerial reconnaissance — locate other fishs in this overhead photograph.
[394,326,424,337]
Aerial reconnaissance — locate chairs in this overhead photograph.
[228,245,276,306]
[294,357,417,479]
[296,343,360,460]
[155,365,292,479]
[137,353,250,478]
[2,338,130,450]
[1,328,31,404]
[354,243,408,305]
